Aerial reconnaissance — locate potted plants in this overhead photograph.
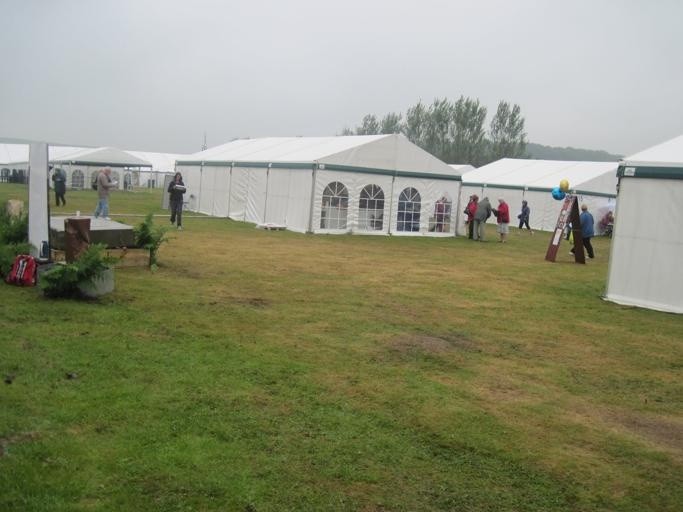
[35,238,128,302]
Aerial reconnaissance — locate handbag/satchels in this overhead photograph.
[463,205,471,215]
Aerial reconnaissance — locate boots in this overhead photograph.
[514,228,535,237]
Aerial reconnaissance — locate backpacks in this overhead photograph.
[4,253,39,289]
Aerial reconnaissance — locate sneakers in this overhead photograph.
[497,238,509,244]
[104,216,112,221]
[568,251,575,257]
[169,221,184,232]
[585,255,595,259]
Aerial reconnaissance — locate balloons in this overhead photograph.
[560,180,569,192]
[552,186,565,200]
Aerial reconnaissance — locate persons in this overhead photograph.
[52,168,67,207]
[564,214,572,241]
[517,201,535,236]
[92,167,117,219]
[569,206,594,259]
[599,211,614,237]
[168,172,186,229]
[473,197,492,242]
[430,198,448,232]
[496,198,510,243]
[467,196,480,240]
